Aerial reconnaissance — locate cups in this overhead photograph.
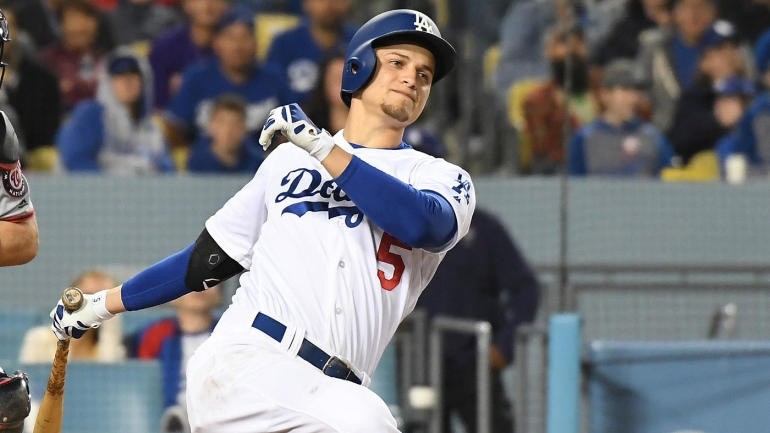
[726,153,745,186]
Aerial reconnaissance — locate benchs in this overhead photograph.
[0,305,396,433]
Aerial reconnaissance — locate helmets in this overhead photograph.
[340,9,456,108]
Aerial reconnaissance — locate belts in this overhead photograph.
[252,313,361,384]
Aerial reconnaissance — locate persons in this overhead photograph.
[0,11,40,433]
[0,0,770,181]
[398,131,538,433]
[46,11,475,433]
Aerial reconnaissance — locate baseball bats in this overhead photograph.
[34,285,85,433]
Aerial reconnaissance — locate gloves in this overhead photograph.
[50,290,116,340]
[259,103,335,163]
